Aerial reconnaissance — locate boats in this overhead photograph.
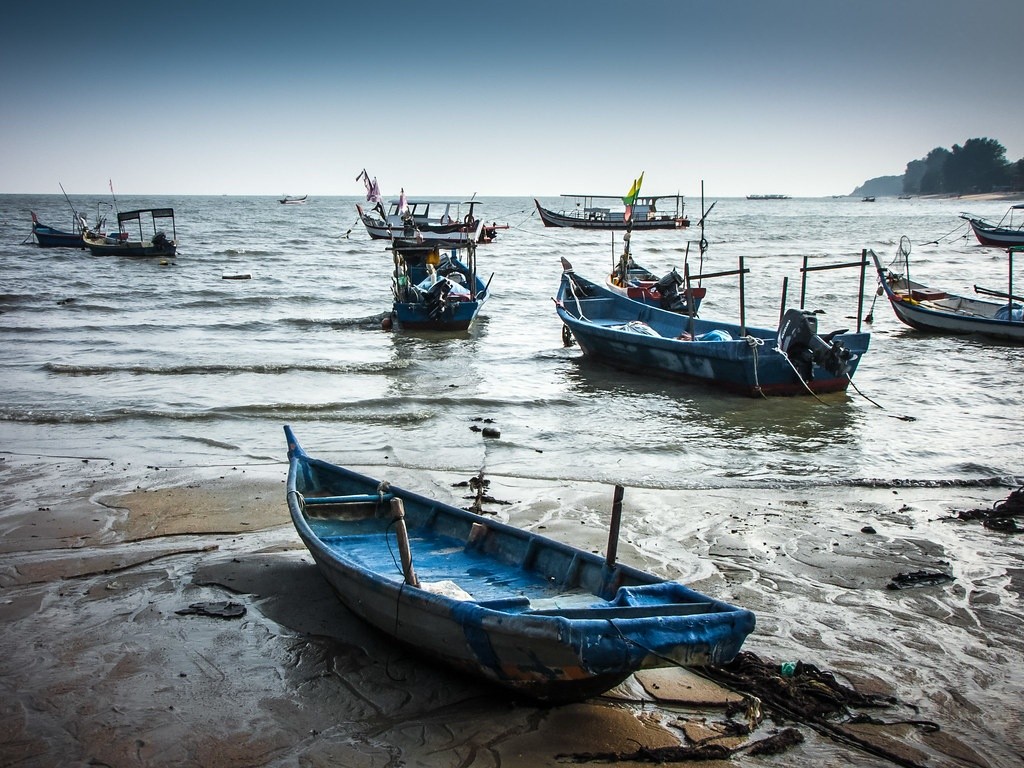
[969,203,1024,247]
[29,182,129,248]
[281,422,758,706]
[384,187,491,334]
[869,247,1024,342]
[547,256,873,398]
[278,195,309,207]
[604,173,718,317]
[78,207,179,259]
[353,166,510,245]
[535,197,695,233]
